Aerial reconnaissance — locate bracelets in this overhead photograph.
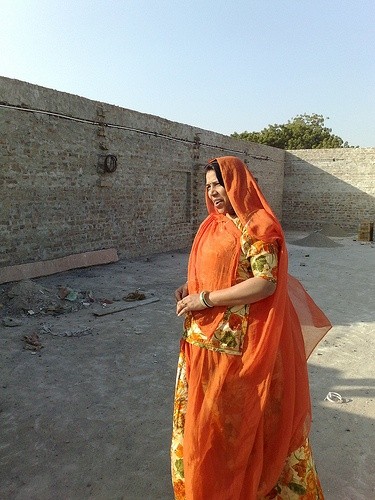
[200,290,213,309]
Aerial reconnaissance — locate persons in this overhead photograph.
[170,156,332,500]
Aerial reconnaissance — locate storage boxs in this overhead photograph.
[358,222,374,242]
